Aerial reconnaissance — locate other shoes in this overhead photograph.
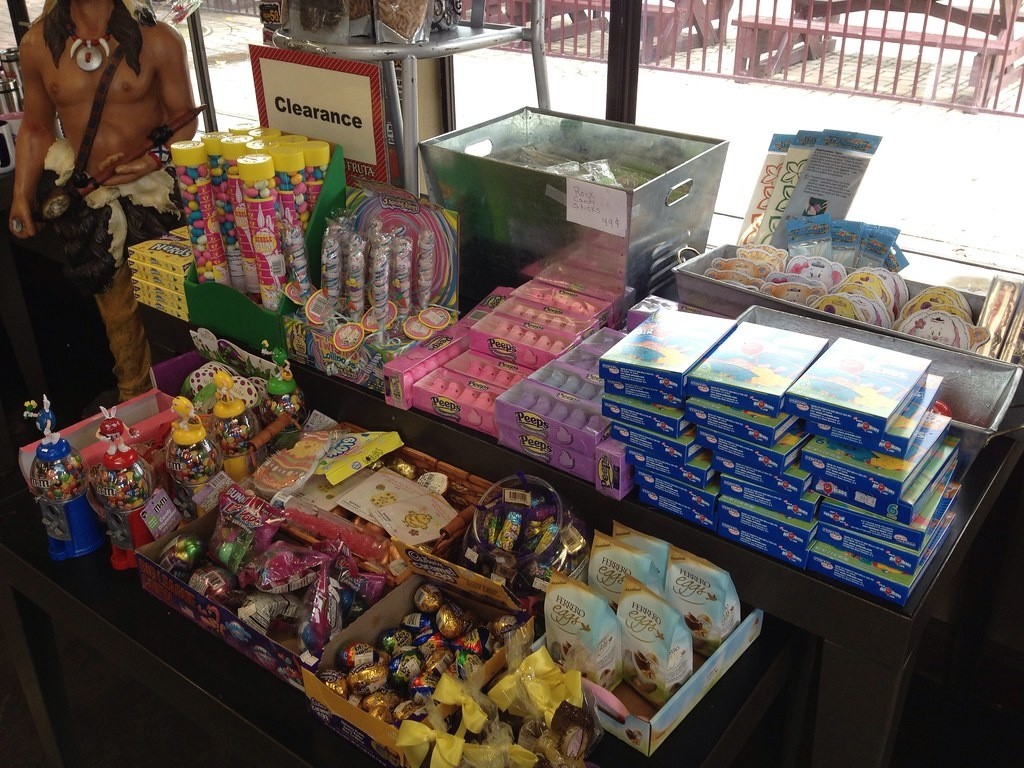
[81,390,120,419]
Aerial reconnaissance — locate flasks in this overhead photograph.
[0,78,22,115]
[0,47,24,99]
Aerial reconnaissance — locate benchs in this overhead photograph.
[462,0,687,63]
[731,13,1016,110]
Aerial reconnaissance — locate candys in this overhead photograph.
[162,443,581,727]
[31,386,303,509]
[172,157,436,344]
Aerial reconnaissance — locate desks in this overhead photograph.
[1,384,822,767]
[134,302,1024,768]
[184,263,1024,768]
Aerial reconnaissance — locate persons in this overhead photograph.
[7,1,196,400]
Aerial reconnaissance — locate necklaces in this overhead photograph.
[65,14,111,71]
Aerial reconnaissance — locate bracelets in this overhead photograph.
[147,144,172,171]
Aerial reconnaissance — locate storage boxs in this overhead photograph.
[19,108,1024,768]
